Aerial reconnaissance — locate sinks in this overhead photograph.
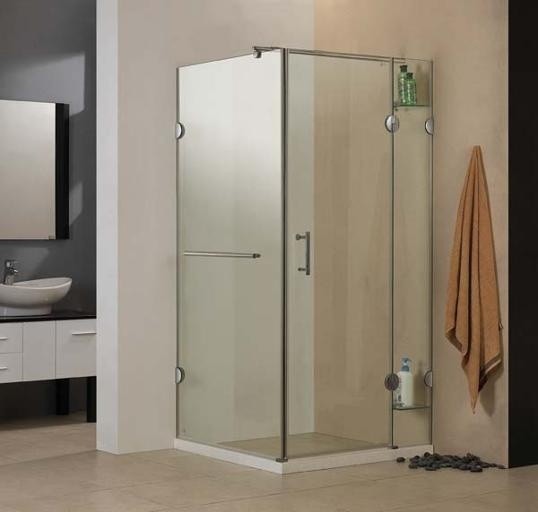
[0,276,72,317]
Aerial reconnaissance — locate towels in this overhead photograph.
[443,144,503,415]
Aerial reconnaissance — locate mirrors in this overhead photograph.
[0,100,69,240]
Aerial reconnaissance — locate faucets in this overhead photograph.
[3,259,20,284]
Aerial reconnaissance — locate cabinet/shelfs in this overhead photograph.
[0,318,98,422]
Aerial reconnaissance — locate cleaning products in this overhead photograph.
[406,71,417,104]
[398,64,408,104]
